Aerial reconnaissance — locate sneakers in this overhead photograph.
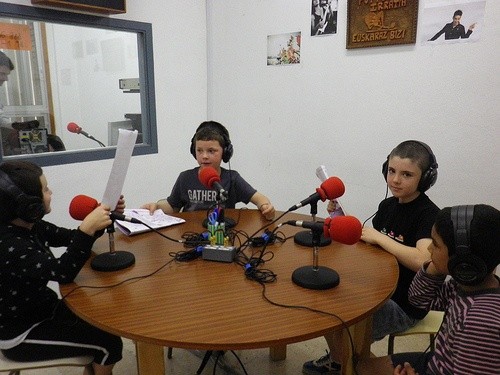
[303,348,342,375]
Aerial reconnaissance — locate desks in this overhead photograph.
[60,209,399,375]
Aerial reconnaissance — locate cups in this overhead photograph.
[208,222,225,245]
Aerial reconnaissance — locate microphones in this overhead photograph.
[67,122,91,137]
[198,167,226,192]
[69,195,142,224]
[288,215,363,244]
[290,177,345,211]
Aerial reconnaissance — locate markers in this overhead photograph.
[214,208,223,225]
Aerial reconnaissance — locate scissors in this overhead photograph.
[208,212,217,225]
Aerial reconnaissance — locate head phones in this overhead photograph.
[381,139,439,193]
[447,203,489,285]
[1,170,45,222]
[190,122,234,161]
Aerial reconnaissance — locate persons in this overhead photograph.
[350,204,500,375]
[427,10,476,40]
[139,115,277,222]
[303,139,445,375]
[0,160,125,375]
[311,0,333,36]
[0,51,19,148]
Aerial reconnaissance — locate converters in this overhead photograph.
[204,244,236,262]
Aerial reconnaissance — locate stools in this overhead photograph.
[0,351,92,375]
[388,310,445,355]
